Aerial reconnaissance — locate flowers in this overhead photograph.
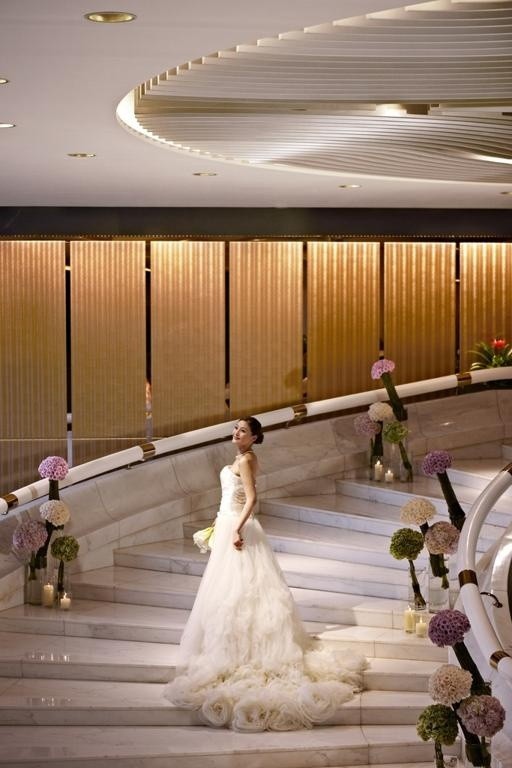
[392,448,512,767]
[12,456,79,609]
[191,525,214,553]
[354,358,414,482]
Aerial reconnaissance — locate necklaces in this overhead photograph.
[233,449,256,458]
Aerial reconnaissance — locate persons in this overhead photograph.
[166,414,313,734]
